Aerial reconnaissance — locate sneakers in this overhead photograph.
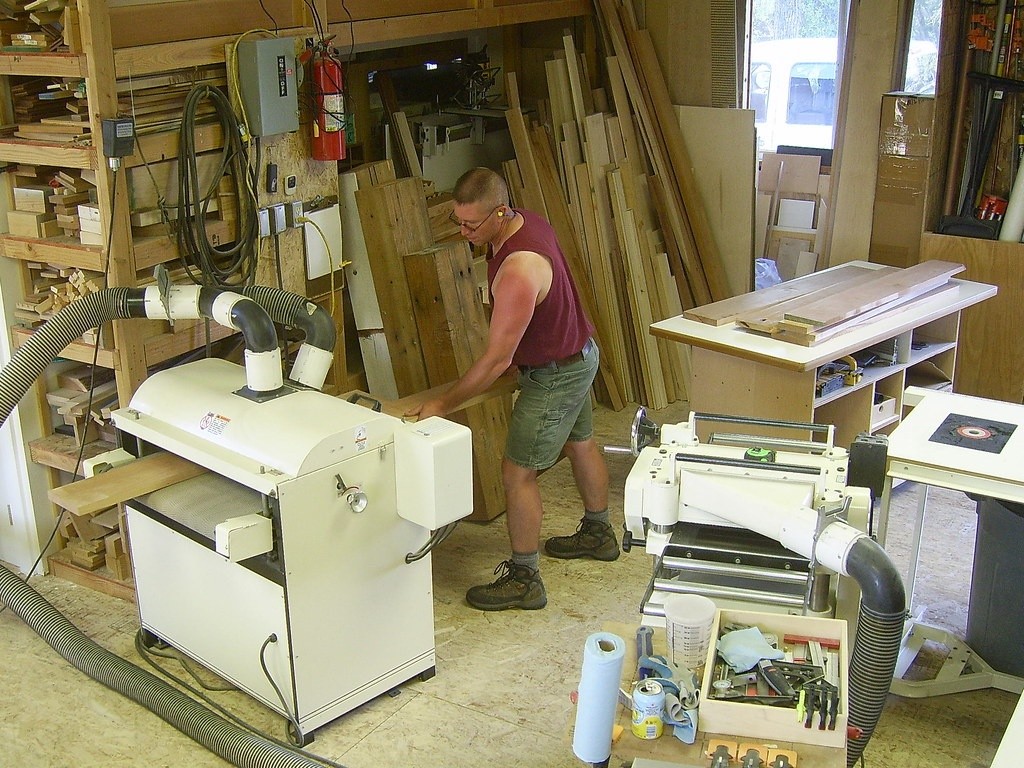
[544,516,620,561]
[466,560,547,612]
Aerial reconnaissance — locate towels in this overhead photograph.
[661,686,700,745]
[715,625,785,674]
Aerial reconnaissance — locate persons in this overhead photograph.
[402,167,621,610]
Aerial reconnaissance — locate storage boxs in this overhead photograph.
[867,91,936,266]
[698,608,853,750]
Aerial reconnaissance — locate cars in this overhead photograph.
[743,37,937,162]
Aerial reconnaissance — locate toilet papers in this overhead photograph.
[572,632,627,764]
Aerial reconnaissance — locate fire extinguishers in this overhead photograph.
[308,34,346,160]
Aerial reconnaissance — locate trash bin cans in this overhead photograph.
[966,495,1024,678]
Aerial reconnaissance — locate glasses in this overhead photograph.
[448,203,505,232]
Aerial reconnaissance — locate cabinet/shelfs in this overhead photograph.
[648,257,999,449]
[0,0,603,606]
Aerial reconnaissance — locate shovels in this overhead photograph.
[940,71,995,240]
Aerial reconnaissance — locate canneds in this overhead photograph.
[631,679,665,740]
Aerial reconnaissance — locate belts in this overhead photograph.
[517,340,592,371]
